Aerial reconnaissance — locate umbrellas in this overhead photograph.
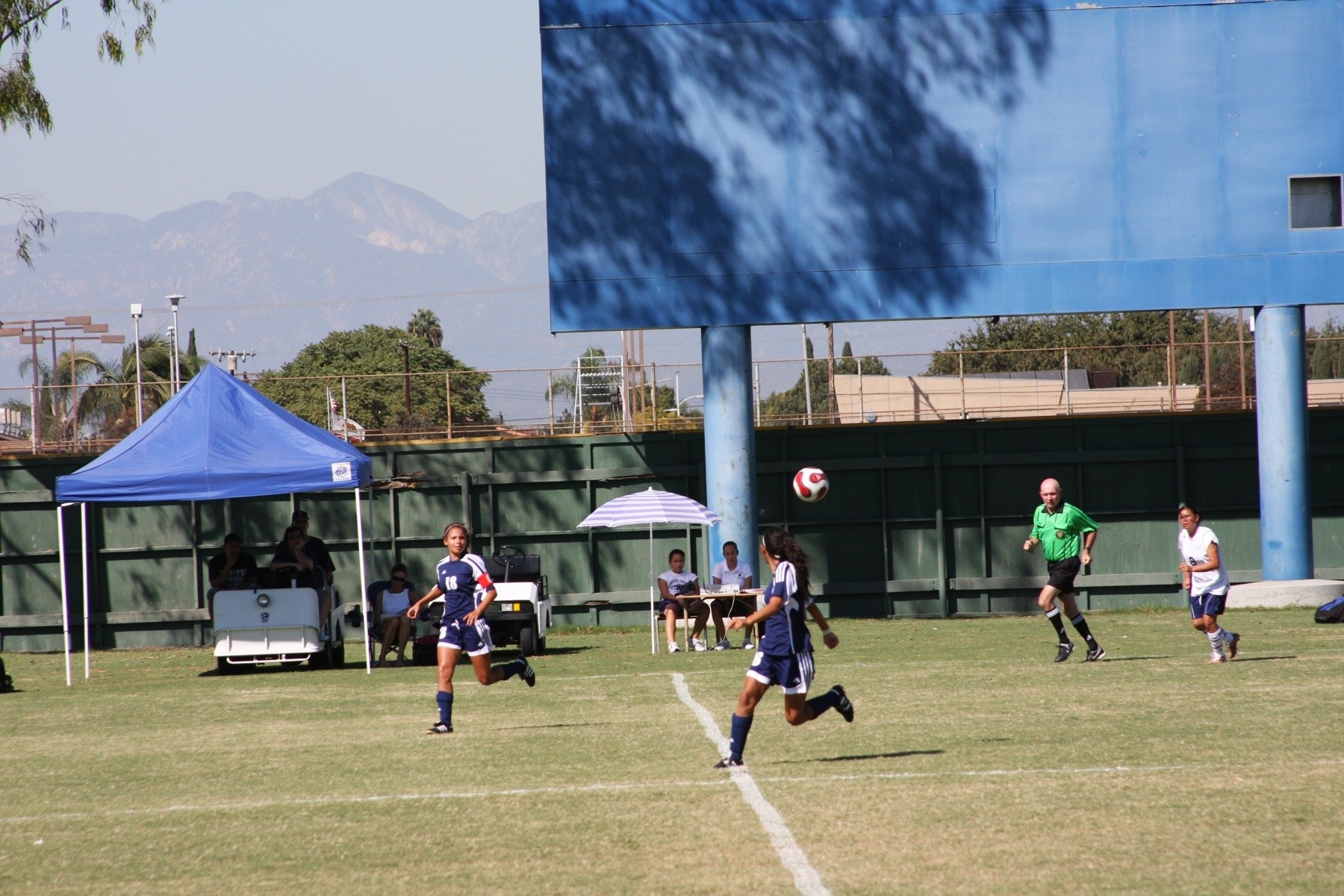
[575,487,724,654]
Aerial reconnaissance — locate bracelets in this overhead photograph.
[822,628,833,636]
[1084,547,1091,550]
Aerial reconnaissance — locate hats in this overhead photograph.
[293,511,309,520]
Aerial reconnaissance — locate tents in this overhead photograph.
[55,361,373,675]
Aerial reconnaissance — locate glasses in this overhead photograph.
[295,519,307,524]
[391,575,406,583]
[287,535,302,540]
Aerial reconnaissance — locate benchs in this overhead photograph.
[481,555,540,581]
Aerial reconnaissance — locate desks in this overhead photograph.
[676,594,768,652]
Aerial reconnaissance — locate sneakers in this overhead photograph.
[829,685,853,722]
[1080,645,1106,663]
[743,641,754,649]
[715,641,730,651]
[1054,640,1076,662]
[1207,656,1227,664]
[713,758,743,768]
[1227,633,1240,658]
[668,642,681,653]
[426,721,453,734]
[688,636,705,652]
[516,656,535,687]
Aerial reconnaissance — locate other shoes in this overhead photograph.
[395,660,405,668]
[377,659,385,668]
[319,634,329,641]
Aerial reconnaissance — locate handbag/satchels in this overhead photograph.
[1314,595,1344,623]
[679,581,696,595]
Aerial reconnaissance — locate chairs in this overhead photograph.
[655,592,707,652]
[368,581,417,665]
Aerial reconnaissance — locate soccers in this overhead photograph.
[792,466,830,504]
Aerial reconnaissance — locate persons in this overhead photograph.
[373,563,420,667]
[714,526,855,771]
[657,549,710,653]
[710,541,754,650]
[1023,478,1106,663]
[270,511,335,642]
[206,533,262,621]
[1176,504,1240,664]
[406,524,535,735]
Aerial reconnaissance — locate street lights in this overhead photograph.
[130,303,142,428]
[164,293,186,394]
